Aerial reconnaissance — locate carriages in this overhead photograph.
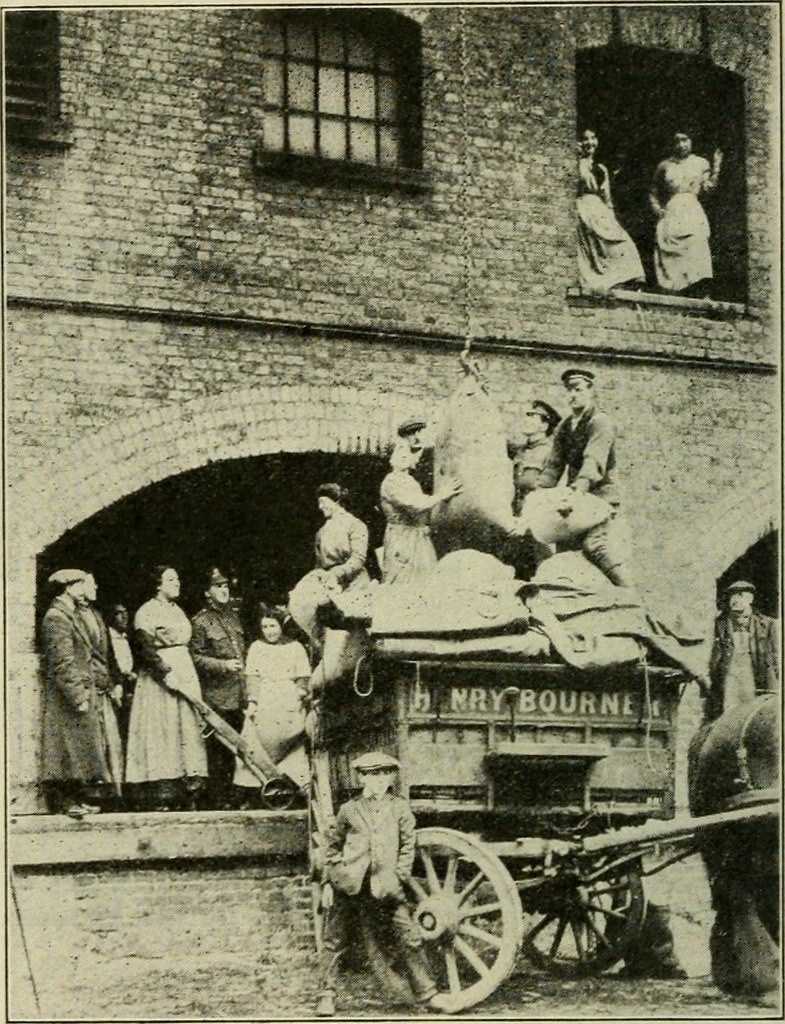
[301,652,782,1014]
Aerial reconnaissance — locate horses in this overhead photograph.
[687,688,782,1010]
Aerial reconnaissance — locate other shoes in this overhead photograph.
[315,996,335,1017]
[78,802,101,814]
[417,993,464,1015]
[54,804,87,817]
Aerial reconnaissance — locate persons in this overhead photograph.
[314,750,469,1017]
[647,127,724,301]
[505,369,633,590]
[375,415,465,584]
[576,125,646,298]
[701,580,782,720]
[39,565,318,818]
[314,482,370,594]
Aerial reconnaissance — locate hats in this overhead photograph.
[194,568,229,586]
[561,369,595,384]
[728,580,755,592]
[48,569,86,584]
[398,419,426,436]
[350,752,401,772]
[526,400,562,426]
